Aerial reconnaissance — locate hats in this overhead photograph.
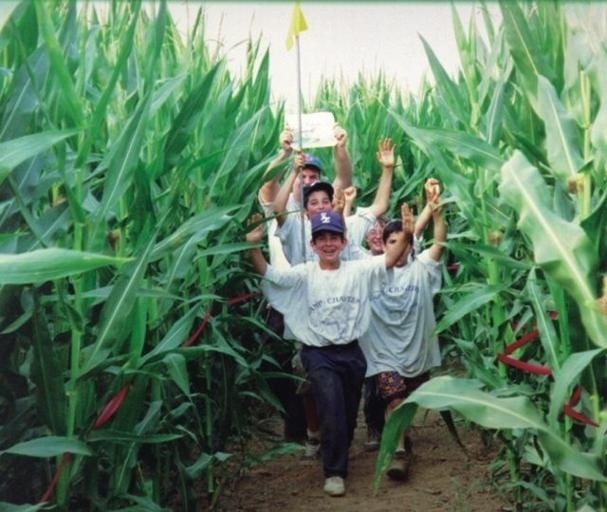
[303,180,335,198]
[311,212,345,234]
[305,156,324,170]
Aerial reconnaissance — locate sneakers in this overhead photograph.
[364,433,380,452]
[302,429,321,460]
[386,449,410,481]
[323,476,346,497]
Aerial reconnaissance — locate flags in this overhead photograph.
[286,0,309,51]
